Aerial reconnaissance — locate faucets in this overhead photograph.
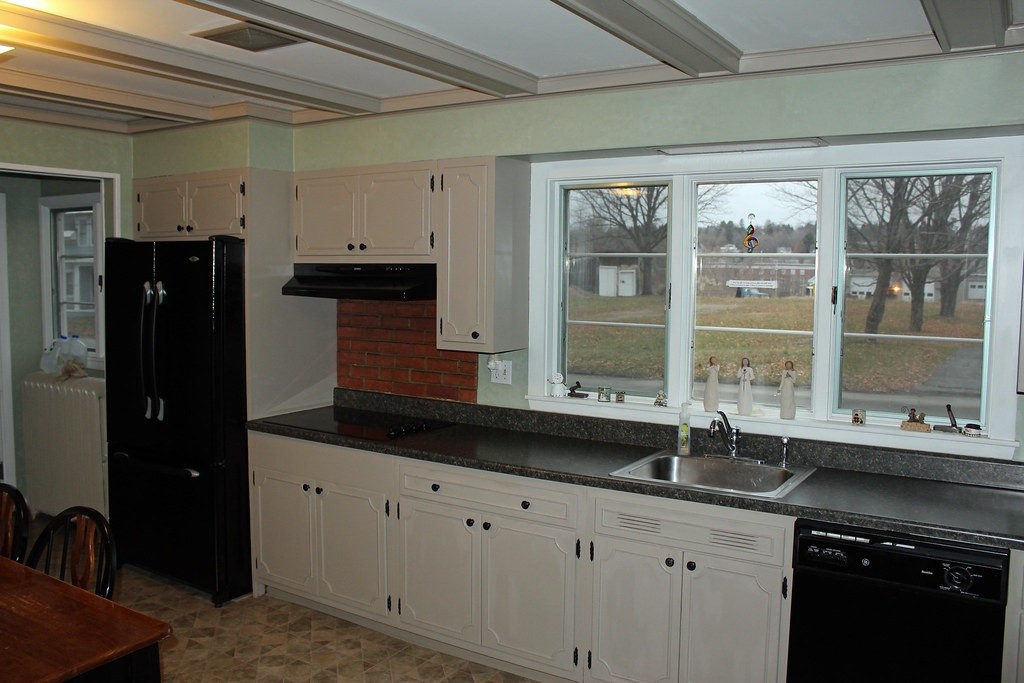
[708,410,740,456]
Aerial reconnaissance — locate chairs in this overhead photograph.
[26,506,119,600]
[0,482,30,564]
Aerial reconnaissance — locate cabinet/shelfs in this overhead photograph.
[290,156,532,353]
[247,402,825,683]
[135,165,249,240]
[100,235,252,610]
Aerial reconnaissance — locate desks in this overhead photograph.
[0,555,174,683]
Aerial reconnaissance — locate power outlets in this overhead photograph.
[490,361,513,385]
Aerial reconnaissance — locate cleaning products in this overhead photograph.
[677,403,692,456]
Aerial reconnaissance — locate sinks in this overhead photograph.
[609,450,817,499]
